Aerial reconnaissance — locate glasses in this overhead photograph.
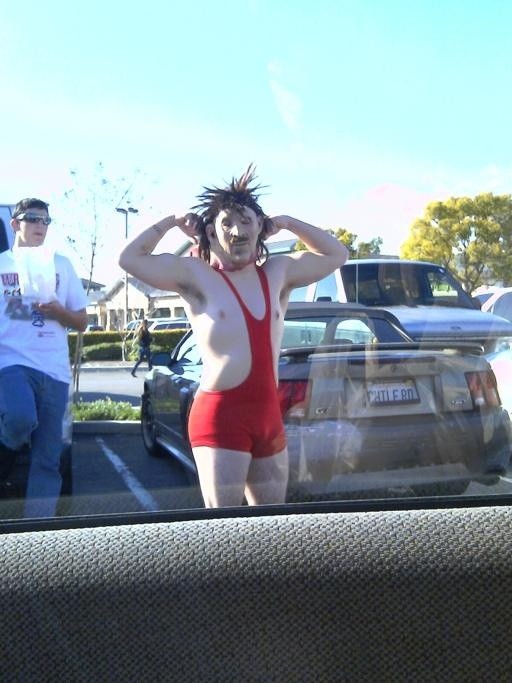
[15,212,51,226]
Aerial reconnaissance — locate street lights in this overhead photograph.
[112,202,139,328]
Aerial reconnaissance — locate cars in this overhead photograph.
[68,323,105,332]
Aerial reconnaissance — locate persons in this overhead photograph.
[0,197,90,521]
[129,318,155,379]
[116,159,349,510]
